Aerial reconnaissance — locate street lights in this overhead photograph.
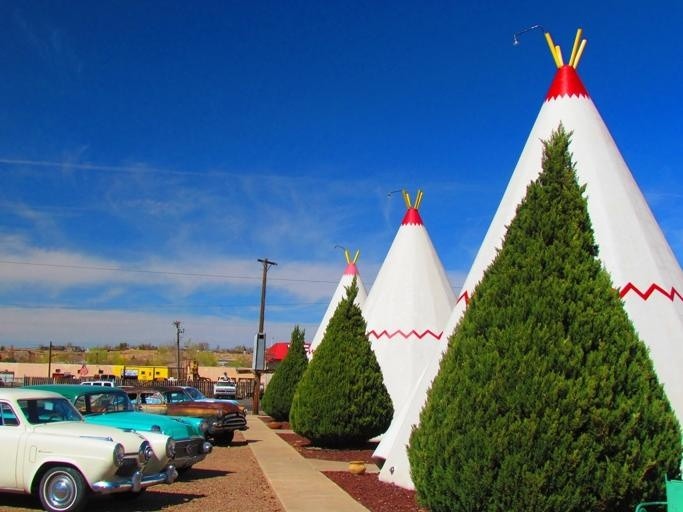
[173,319,184,380]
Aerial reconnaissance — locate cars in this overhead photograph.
[214,377,238,399]
[0,385,213,474]
[130,386,242,406]
[0,387,179,511]
[77,387,249,446]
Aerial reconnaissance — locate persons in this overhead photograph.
[223,372,229,381]
[192,360,200,380]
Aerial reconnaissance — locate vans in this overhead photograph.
[78,381,115,389]
